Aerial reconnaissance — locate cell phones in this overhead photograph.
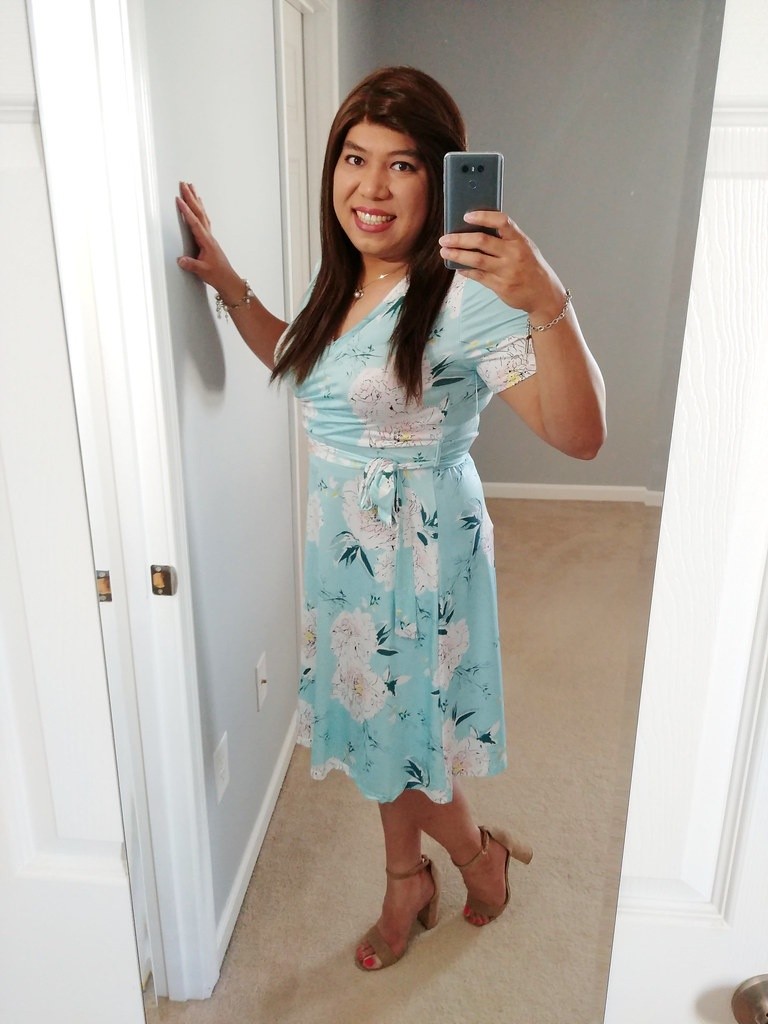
[444,152,504,269]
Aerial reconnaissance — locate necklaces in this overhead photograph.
[354,260,410,300]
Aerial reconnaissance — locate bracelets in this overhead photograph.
[524,287,572,354]
[215,279,255,324]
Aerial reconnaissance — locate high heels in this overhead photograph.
[450,820,532,926]
[354,852,444,971]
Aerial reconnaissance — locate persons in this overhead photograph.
[177,66,606,972]
